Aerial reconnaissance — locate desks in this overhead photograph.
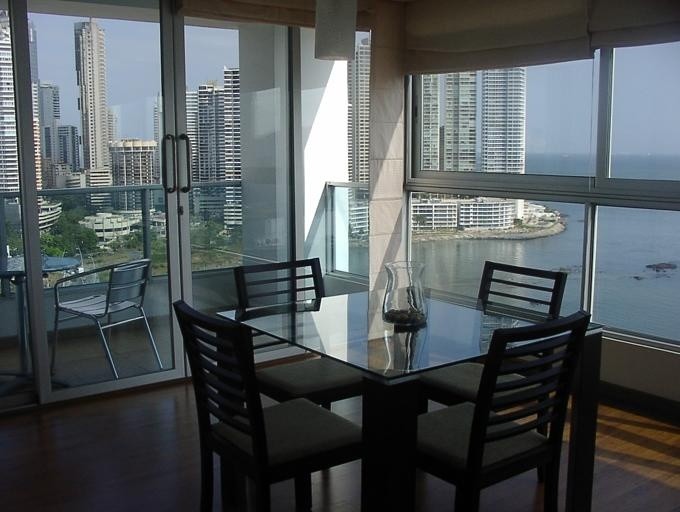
[0,256,81,377]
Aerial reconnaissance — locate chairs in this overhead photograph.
[173,299,366,512]
[415,309,591,512]
[50,259,163,380]
[233,258,365,413]
[420,261,568,484]
